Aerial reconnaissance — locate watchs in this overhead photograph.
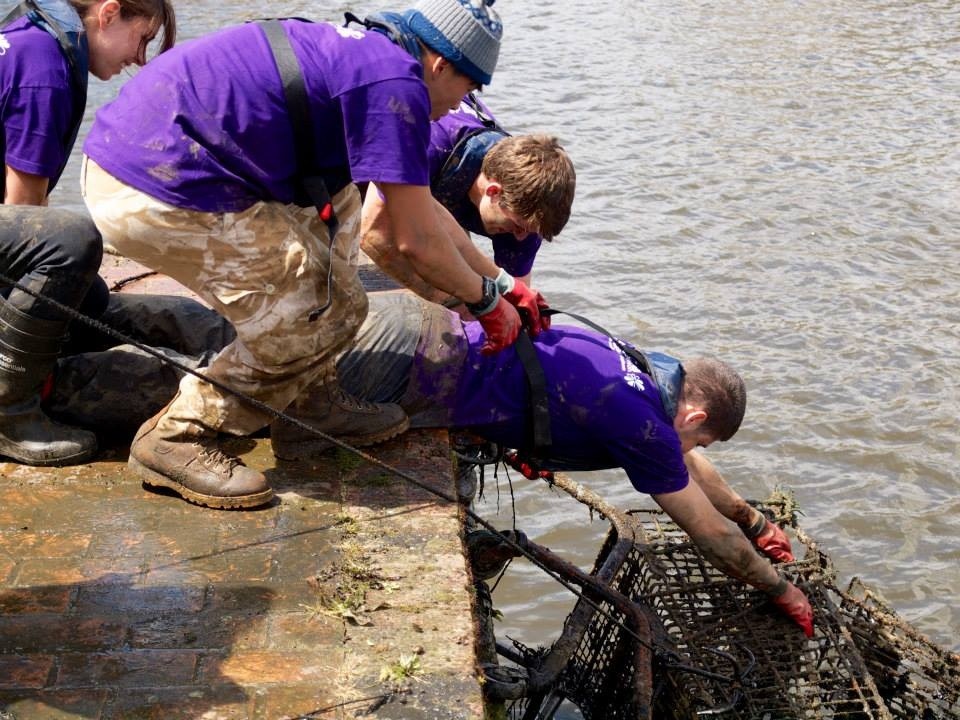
[464,275,496,312]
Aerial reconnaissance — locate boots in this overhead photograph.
[0,297,98,468]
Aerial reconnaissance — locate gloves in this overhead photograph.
[495,268,552,339]
[465,279,523,355]
[761,578,814,639]
[746,510,795,562]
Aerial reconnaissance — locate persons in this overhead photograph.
[41,292,814,639]
[79,0,504,512]
[0,0,177,467]
[360,92,576,320]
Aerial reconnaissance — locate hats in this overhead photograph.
[404,0,503,87]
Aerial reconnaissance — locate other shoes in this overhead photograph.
[271,381,411,458]
[126,398,275,510]
